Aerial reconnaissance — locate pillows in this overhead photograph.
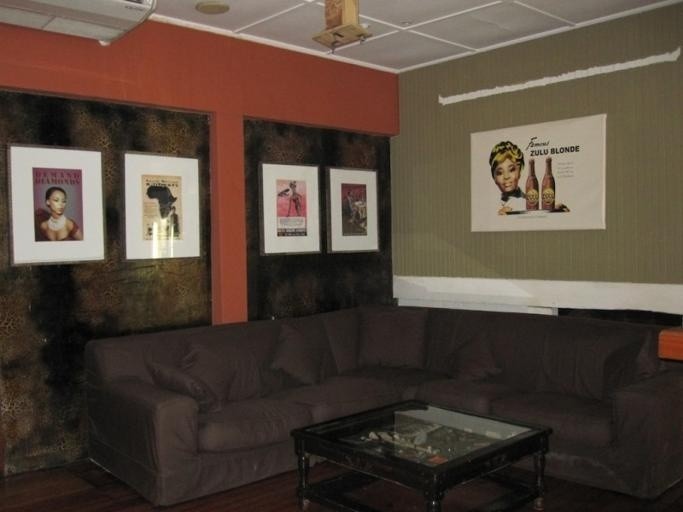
[360,301,431,371]
[175,324,264,402]
[144,356,217,416]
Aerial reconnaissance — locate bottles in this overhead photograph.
[540,157,556,210]
[524,159,539,210]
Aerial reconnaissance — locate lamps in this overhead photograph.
[0,1,156,47]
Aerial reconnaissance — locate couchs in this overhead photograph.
[77,298,683,510]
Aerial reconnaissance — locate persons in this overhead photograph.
[486,141,568,215]
[342,190,361,230]
[40,186,83,241]
[285,182,303,216]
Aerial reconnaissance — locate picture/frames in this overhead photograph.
[5,140,105,267]
[323,164,382,254]
[119,147,202,262]
[256,160,322,257]
